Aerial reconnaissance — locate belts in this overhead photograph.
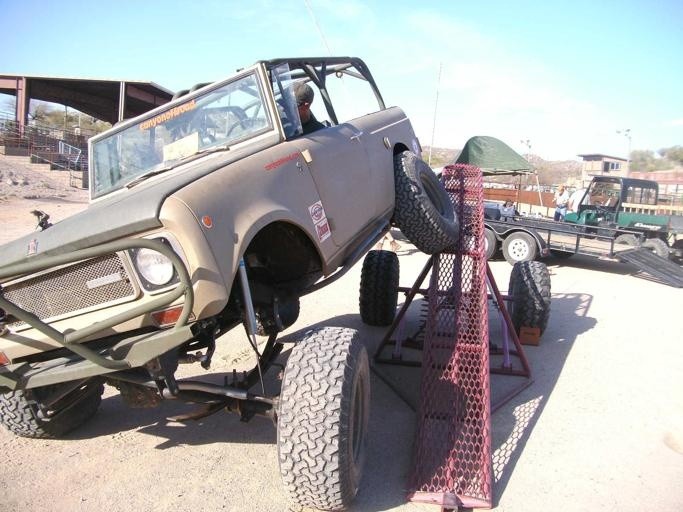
[557,205,566,208]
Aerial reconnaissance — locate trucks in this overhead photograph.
[564,176,683,260]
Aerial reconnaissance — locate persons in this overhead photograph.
[499,198,517,223]
[569,180,592,216]
[553,185,568,221]
[276,80,324,141]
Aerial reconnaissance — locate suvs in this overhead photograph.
[0,57,461,512]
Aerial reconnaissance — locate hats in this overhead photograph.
[275,82,313,110]
